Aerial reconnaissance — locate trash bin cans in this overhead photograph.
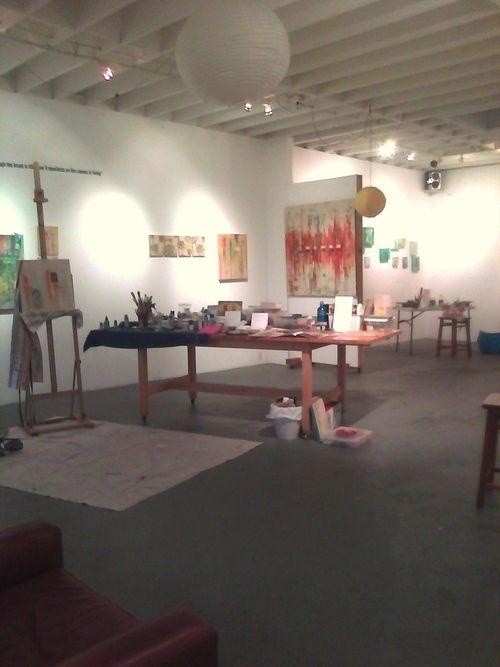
[270,396,303,440]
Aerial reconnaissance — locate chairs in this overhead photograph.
[0,517,222,666]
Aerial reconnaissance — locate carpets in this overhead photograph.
[0,415,266,512]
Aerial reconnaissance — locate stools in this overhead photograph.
[437,316,472,360]
[476,392,500,506]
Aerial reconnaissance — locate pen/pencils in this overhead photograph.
[130,291,155,310]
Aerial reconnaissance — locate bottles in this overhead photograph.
[317,301,327,324]
[124,314,129,327]
[103,316,110,327]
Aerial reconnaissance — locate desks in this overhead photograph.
[393,305,475,356]
[90,325,404,438]
